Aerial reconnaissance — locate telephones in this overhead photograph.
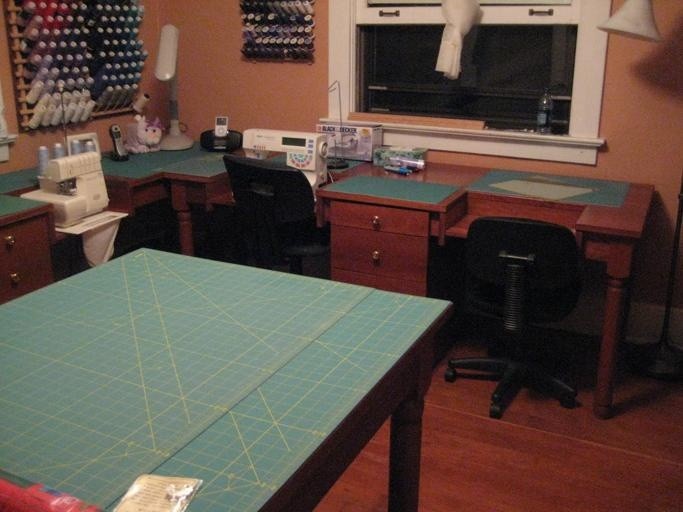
[110,124,129,161]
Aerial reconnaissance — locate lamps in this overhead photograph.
[597,1,661,44]
[154,25,194,151]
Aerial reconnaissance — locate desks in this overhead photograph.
[2,138,394,302]
[0,246,455,512]
[315,146,653,418]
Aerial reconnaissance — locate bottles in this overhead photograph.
[536,87,553,135]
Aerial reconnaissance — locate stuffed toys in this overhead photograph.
[123,114,165,154]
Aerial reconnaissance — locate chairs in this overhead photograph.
[441,214,587,417]
[221,154,315,277]
